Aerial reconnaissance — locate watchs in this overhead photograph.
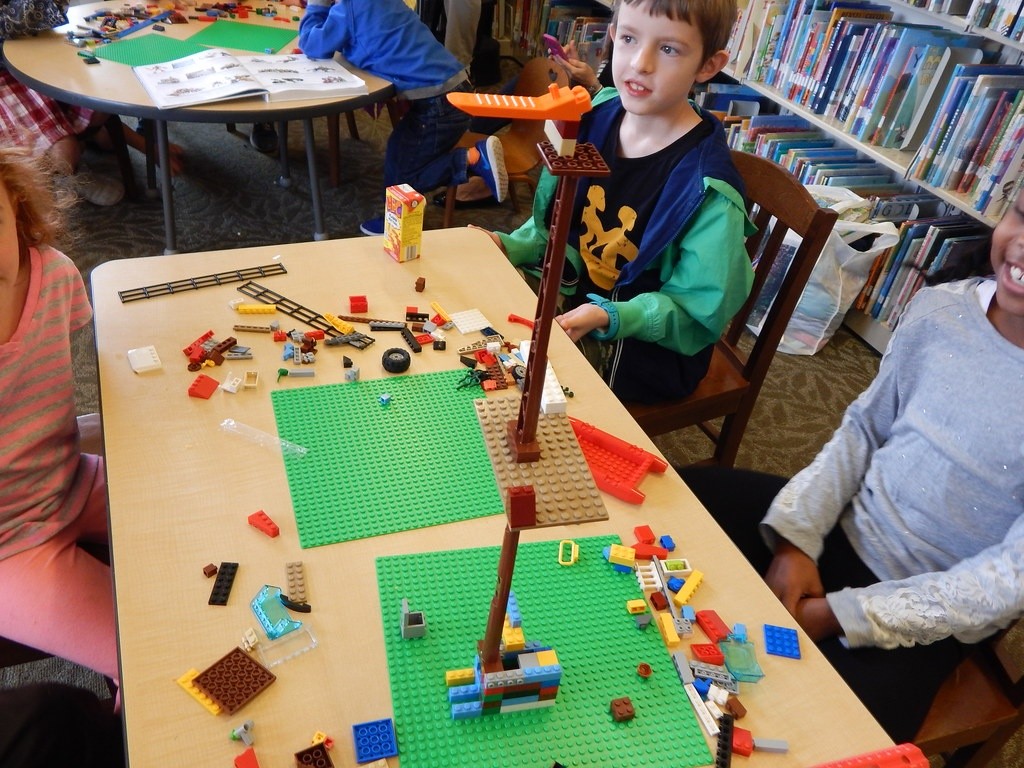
[586,292,620,342]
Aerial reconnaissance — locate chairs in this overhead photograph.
[441,56,571,227]
[621,149,840,468]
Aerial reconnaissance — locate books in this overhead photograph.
[132,47,370,113]
[490,0,1024,334]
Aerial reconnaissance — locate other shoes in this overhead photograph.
[77,169,126,206]
[49,177,77,210]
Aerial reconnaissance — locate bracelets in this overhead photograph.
[587,80,600,94]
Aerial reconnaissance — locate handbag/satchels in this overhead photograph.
[744,183,900,356]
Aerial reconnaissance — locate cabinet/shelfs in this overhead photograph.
[495,0,1024,354]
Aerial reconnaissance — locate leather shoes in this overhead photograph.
[432,185,498,208]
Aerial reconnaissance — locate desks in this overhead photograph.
[89,227,895,768]
[3,0,396,255]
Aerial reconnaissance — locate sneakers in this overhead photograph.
[250,121,279,152]
[360,215,384,237]
[471,136,509,203]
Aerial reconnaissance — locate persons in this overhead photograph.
[673,172,1024,749]
[432,16,695,208]
[0,143,124,717]
[466,0,758,407]
[1,1,509,235]
